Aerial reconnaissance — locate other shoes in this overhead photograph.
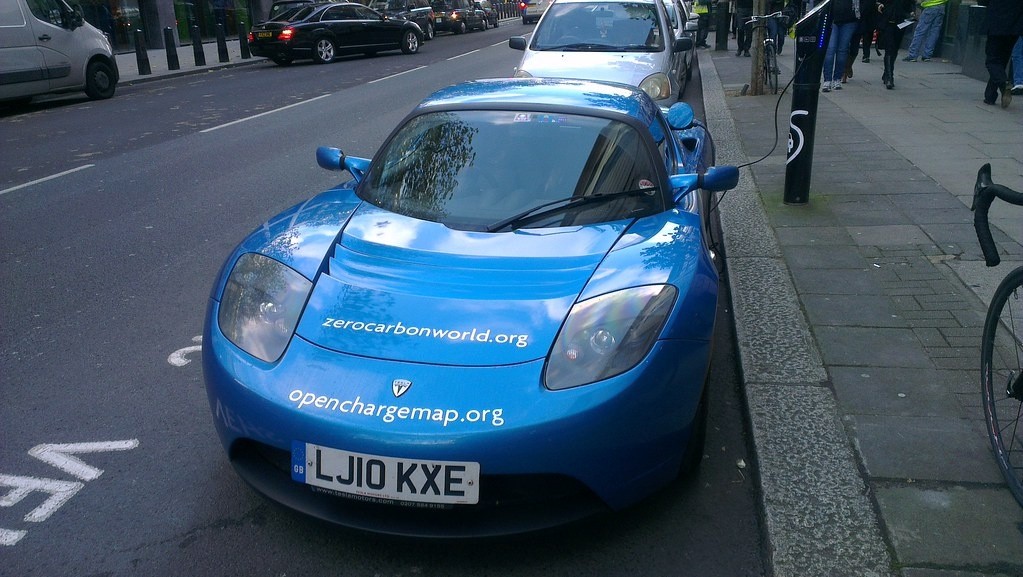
[822,80,832,92]
[846,68,853,78]
[1001,90,1012,108]
[697,40,712,48]
[776,47,782,54]
[840,73,846,83]
[832,79,843,90]
[983,91,998,105]
[743,49,750,57]
[734,49,742,56]
[921,57,931,62]
[862,56,871,63]
[902,56,917,62]
[1011,84,1023,94]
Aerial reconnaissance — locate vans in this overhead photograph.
[1,0,120,109]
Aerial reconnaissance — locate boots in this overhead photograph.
[882,55,895,89]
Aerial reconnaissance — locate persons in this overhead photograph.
[694,0,1023,107]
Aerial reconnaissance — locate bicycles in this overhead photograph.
[744,11,790,94]
[971,161,1022,510]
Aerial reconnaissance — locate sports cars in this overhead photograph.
[201,77,740,552]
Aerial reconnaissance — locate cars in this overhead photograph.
[367,0,501,40]
[520,1,552,25]
[652,0,700,81]
[249,2,425,66]
[509,1,694,119]
